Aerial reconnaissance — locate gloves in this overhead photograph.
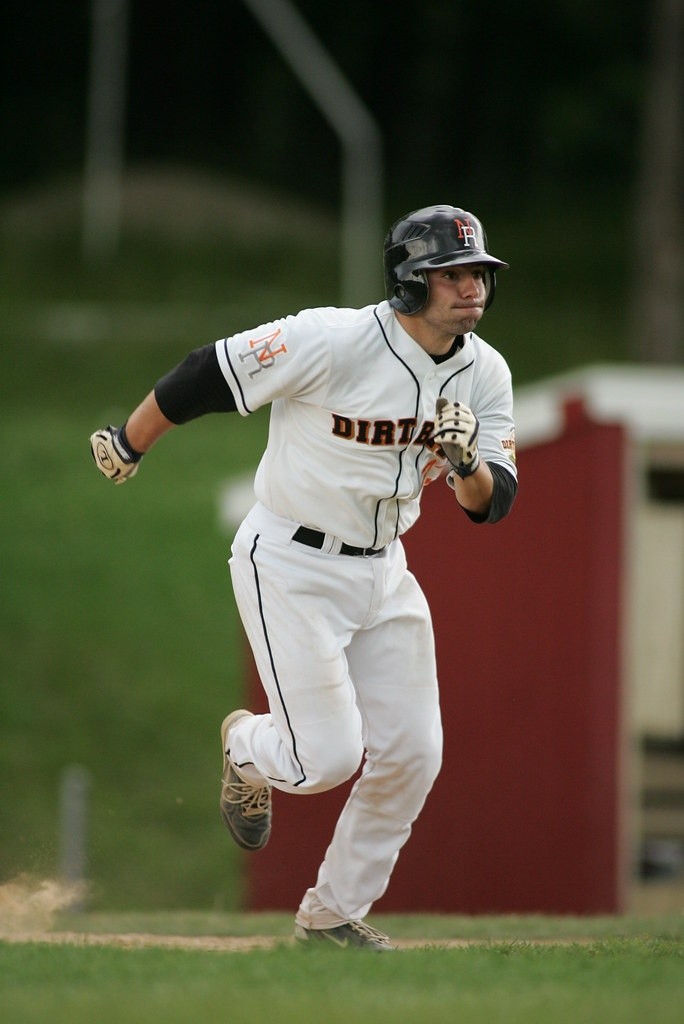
[429,397,480,479]
[89,418,144,486]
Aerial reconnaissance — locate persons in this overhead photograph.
[90,204,519,948]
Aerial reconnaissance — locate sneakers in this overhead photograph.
[220,709,271,854]
[289,917,394,954]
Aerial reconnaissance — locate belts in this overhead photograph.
[291,526,385,558]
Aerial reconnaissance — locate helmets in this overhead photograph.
[383,205,509,316]
